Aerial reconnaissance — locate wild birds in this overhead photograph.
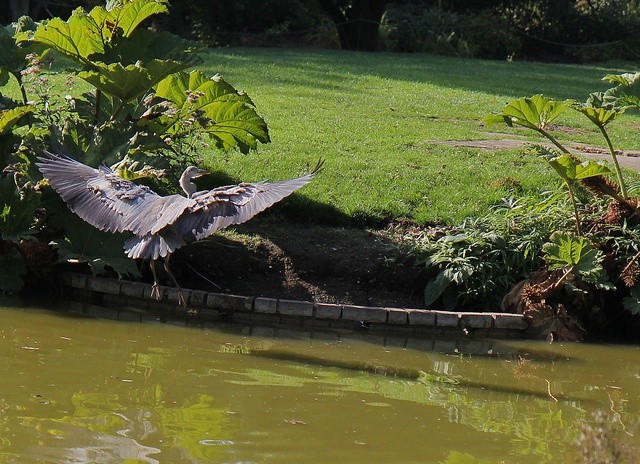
[34,147,326,309]
